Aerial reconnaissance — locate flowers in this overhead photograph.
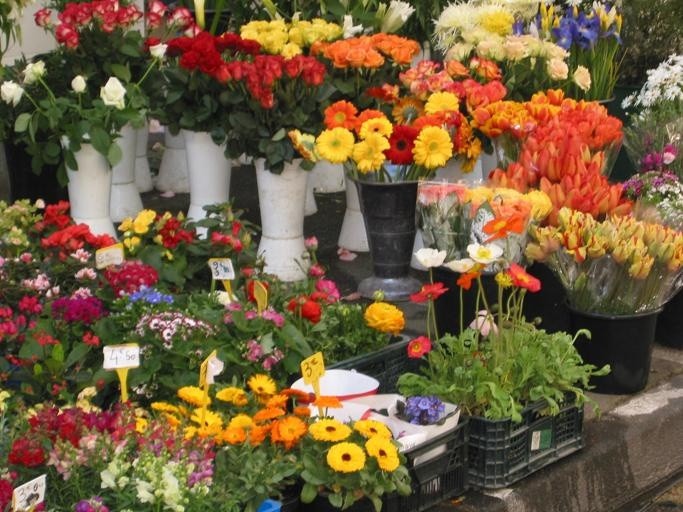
[1,0,682,511]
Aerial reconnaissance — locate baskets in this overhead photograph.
[282,332,411,414]
[246,320,588,511]
[467,390,586,489]
[248,413,468,512]
